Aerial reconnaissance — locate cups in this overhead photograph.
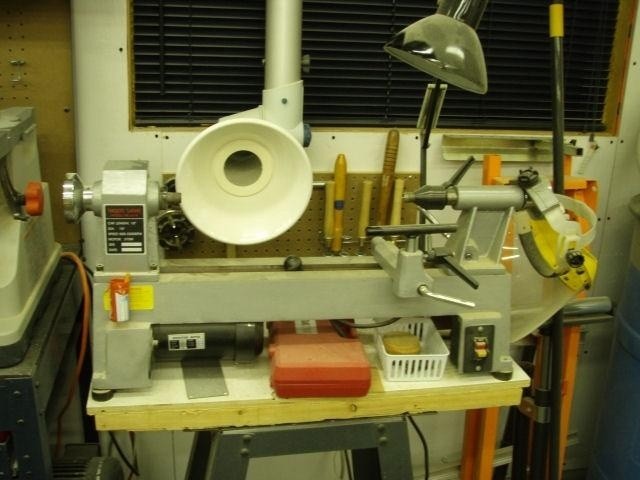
[111,275,132,323]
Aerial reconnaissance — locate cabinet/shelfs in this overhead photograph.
[1,241,102,480]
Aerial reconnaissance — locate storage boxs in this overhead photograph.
[267,333,371,398]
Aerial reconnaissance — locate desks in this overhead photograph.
[85,328,531,480]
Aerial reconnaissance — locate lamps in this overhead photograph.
[382,13,486,253]
